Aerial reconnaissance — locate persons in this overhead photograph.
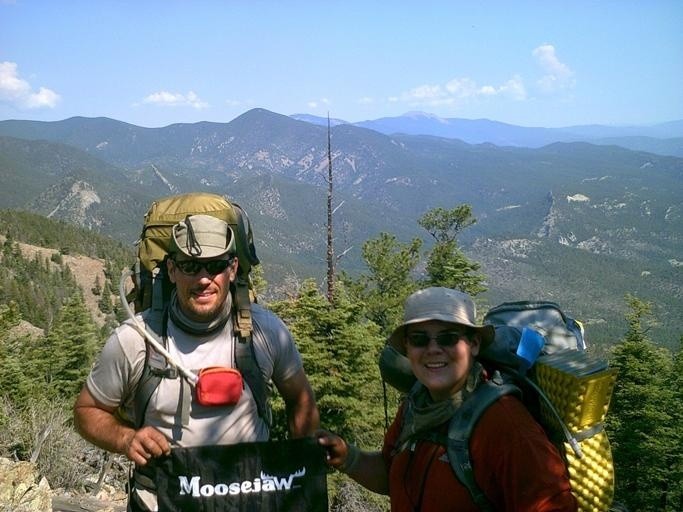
[315,287,578,512]
[74,214,320,512]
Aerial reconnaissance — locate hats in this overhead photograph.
[169,215,237,258]
[390,287,495,357]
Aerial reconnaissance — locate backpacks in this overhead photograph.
[483,301,586,355]
[126,192,259,313]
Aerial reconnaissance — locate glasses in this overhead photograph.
[171,258,233,275]
[404,329,478,347]
